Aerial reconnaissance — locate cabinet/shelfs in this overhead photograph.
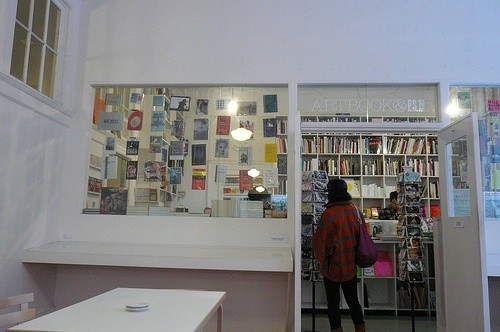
[303,122,440,316]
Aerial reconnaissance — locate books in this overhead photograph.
[302,132,441,280]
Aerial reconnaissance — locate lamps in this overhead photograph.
[247,169,260,178]
[256,185,265,192]
[230,128,253,140]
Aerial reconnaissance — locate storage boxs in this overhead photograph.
[364,267,374,276]
[375,252,393,277]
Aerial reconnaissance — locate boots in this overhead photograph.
[354,321,365,332]
[331,326,343,332]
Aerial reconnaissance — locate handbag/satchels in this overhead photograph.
[351,203,377,268]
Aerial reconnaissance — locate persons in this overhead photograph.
[378,191,399,221]
[314,179,374,332]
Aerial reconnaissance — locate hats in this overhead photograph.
[324,179,347,192]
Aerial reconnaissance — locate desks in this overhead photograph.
[6,287,227,332]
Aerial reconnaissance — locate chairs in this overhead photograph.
[0,292,37,332]
